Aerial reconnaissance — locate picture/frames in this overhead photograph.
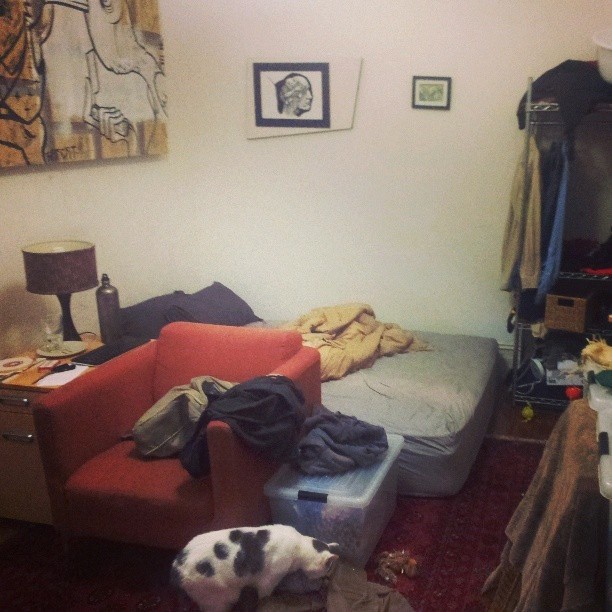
[254,61,331,129]
[410,74,451,109]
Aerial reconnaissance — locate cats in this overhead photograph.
[167,524,340,612]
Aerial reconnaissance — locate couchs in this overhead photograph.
[34,321,321,551]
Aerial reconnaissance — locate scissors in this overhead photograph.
[32,364,75,385]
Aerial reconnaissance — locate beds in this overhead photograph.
[97,276,501,496]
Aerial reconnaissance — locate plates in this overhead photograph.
[37,340,87,358]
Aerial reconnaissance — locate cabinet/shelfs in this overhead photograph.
[1,341,105,528]
[511,77,612,411]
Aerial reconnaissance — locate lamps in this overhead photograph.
[23,240,99,342]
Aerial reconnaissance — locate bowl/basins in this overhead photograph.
[593,28,612,82]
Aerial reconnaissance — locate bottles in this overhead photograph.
[95,274,122,344]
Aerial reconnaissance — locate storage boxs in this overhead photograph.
[544,285,595,334]
[262,434,405,571]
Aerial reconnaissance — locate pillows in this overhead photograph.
[119,291,184,334]
[173,282,263,326]
